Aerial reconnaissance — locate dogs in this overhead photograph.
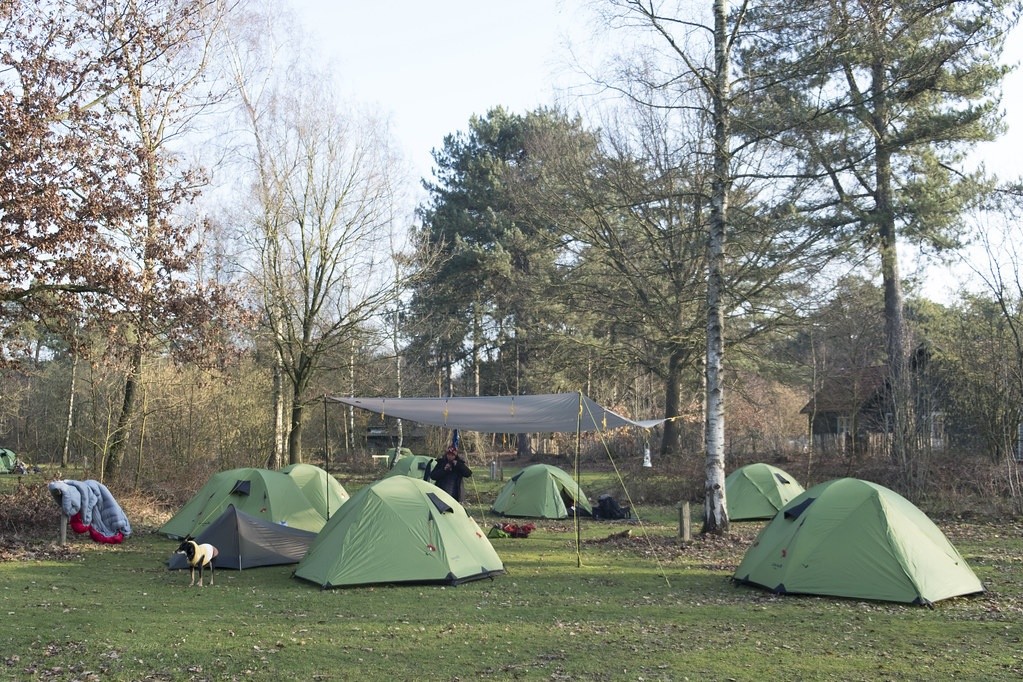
[174,538,219,588]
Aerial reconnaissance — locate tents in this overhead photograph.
[150,466,328,533]
[725,462,806,520]
[320,388,705,568]
[379,455,440,486]
[488,463,595,519]
[288,473,512,587]
[1,446,26,475]
[278,457,351,521]
[728,476,988,610]
[379,446,416,469]
[159,502,319,571]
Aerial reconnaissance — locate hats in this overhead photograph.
[447,446,458,457]
